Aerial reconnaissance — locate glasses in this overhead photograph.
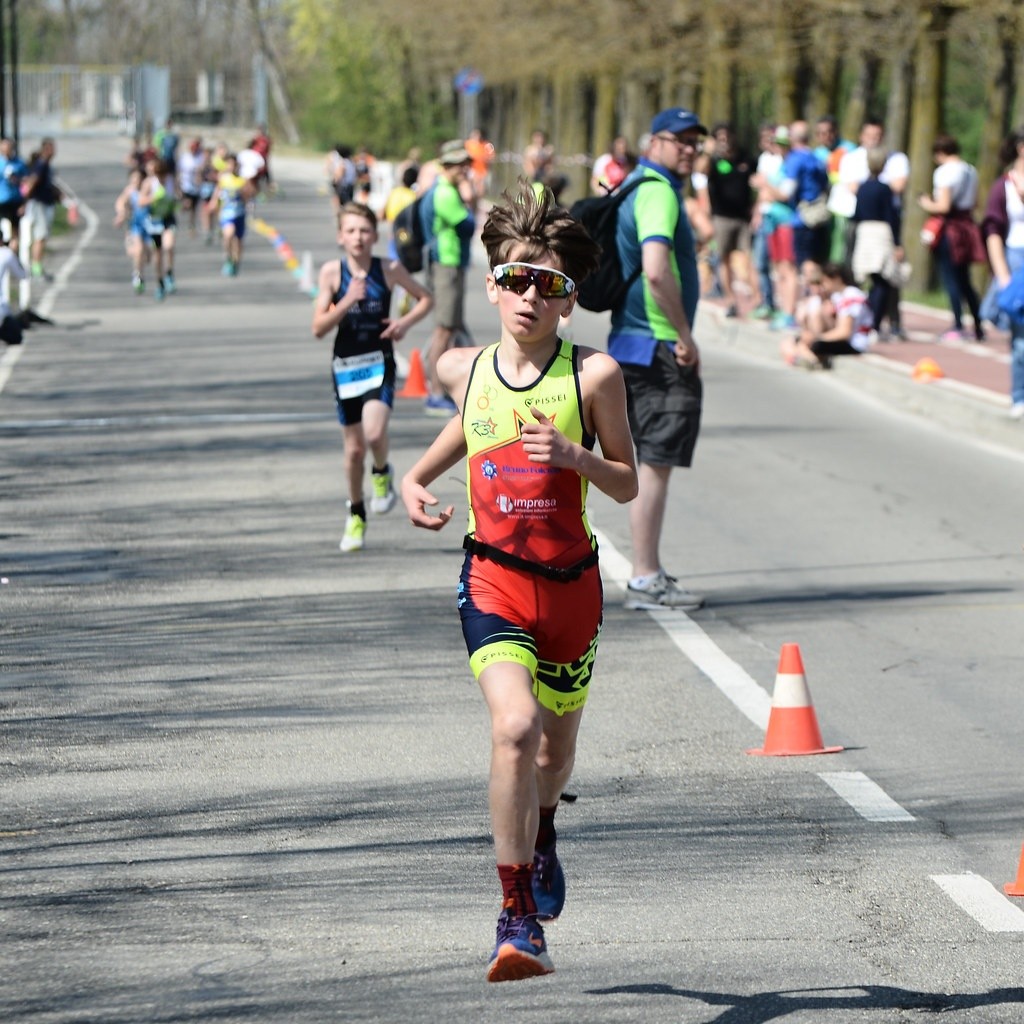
[658,135,706,155]
[493,262,577,299]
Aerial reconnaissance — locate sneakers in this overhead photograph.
[338,513,366,552]
[368,463,395,514]
[486,907,554,982]
[532,822,566,919]
[622,568,704,610]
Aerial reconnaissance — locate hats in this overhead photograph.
[649,107,710,135]
[767,125,790,146]
[439,139,474,167]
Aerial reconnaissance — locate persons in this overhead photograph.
[401,175,639,982]
[917,133,983,343]
[980,125,1024,418]
[591,115,910,372]
[114,122,270,302]
[608,107,705,612]
[0,135,64,346]
[326,128,569,417]
[311,203,434,552]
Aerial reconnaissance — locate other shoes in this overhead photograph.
[154,270,174,304]
[753,305,794,330]
[131,277,142,293]
[424,393,459,416]
[940,324,971,341]
[221,256,237,277]
[725,303,738,317]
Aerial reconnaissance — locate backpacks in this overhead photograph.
[393,194,425,273]
[552,170,682,316]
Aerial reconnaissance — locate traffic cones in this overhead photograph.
[395,349,431,397]
[742,643,849,760]
[295,248,318,292]
[1004,836,1024,896]
